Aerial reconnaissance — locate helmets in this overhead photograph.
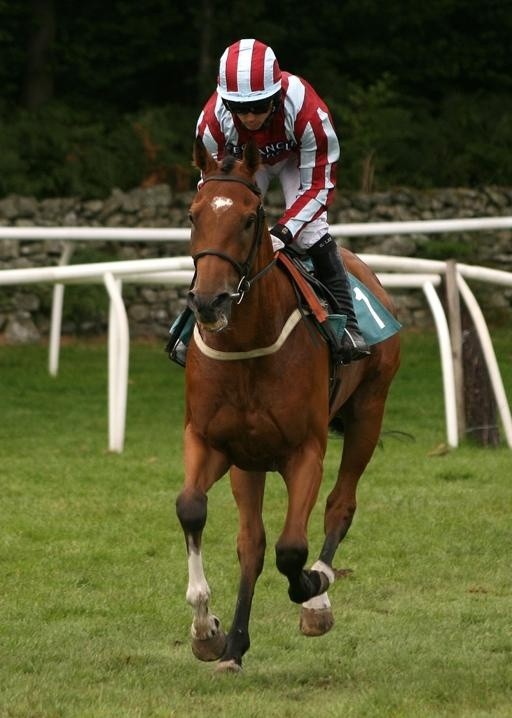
[217,37,283,102]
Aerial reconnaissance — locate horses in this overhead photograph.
[171,132,404,675]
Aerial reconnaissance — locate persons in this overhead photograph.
[166,37,373,365]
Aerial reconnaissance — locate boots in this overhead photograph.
[306,231,371,361]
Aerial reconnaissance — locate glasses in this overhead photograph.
[222,90,281,115]
